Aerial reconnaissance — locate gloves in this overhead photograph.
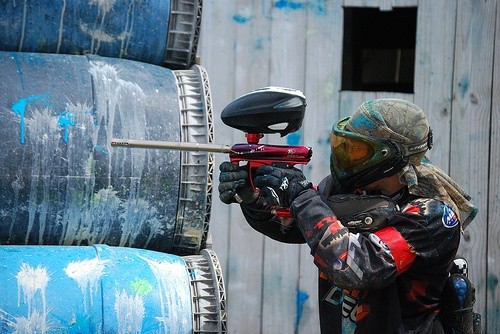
[218,161,270,204]
[253,164,313,208]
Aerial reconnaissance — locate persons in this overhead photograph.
[219,98,479,334]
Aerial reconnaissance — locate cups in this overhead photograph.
[445,273,474,334]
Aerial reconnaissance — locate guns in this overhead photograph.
[111,86,319,227]
